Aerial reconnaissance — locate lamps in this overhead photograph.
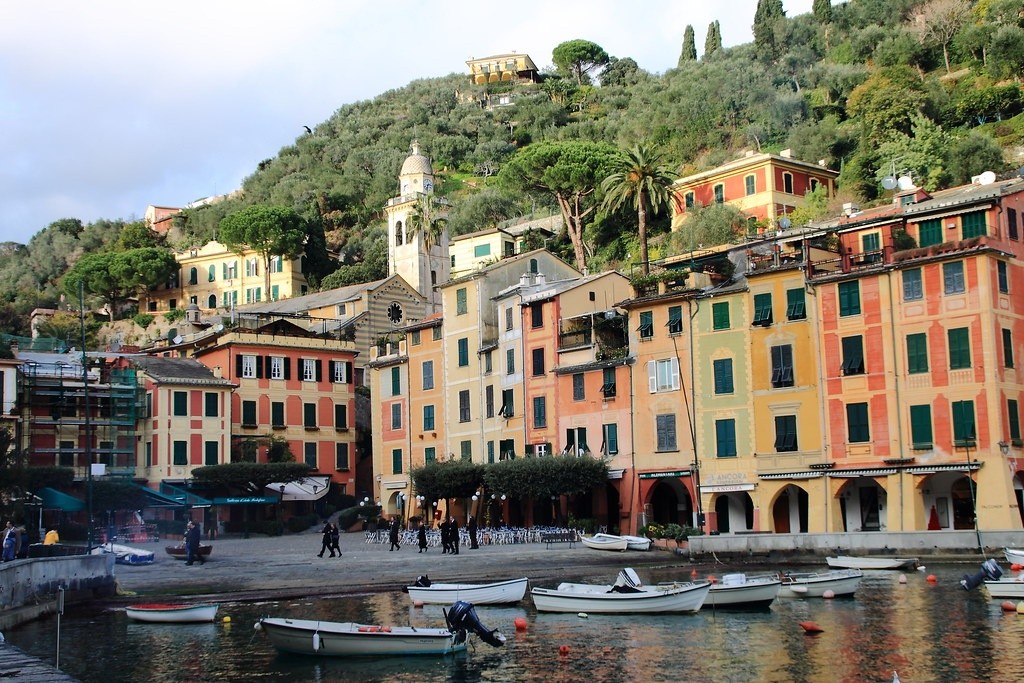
[996,440,1009,455]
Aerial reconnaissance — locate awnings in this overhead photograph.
[30,485,86,512]
[137,477,331,508]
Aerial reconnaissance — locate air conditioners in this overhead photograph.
[972,174,981,184]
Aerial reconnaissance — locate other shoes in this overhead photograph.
[454,552,459,554]
[317,554,322,557]
[449,551,455,554]
[418,550,422,553]
[470,547,478,549]
[425,547,428,552]
[442,552,446,553]
[338,554,342,557]
[396,547,400,550]
[200,559,206,565]
[389,549,393,551]
[185,562,193,566]
[329,553,335,557]
[448,547,450,552]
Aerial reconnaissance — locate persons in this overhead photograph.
[448,516,459,554]
[0,521,29,562]
[183,521,204,566]
[437,517,450,554]
[317,520,335,558]
[389,517,401,552]
[468,516,478,550]
[42,527,60,546]
[417,520,428,553]
[327,523,342,558]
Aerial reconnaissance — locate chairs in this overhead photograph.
[364,525,578,548]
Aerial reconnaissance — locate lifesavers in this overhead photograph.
[358,626,392,633]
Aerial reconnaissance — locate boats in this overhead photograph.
[619,534,652,551]
[1000,546,1024,569]
[399,574,529,606]
[764,567,867,598]
[580,533,628,552]
[101,542,156,564]
[164,541,214,560]
[530,581,714,614]
[958,558,1024,599]
[125,603,219,622]
[826,554,919,569]
[252,600,506,656]
[556,577,783,610]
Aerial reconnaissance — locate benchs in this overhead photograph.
[539,533,575,549]
[27,544,89,558]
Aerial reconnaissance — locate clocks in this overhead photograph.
[423,179,433,191]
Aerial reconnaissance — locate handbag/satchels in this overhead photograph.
[331,535,340,542]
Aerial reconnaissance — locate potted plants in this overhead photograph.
[568,521,575,540]
